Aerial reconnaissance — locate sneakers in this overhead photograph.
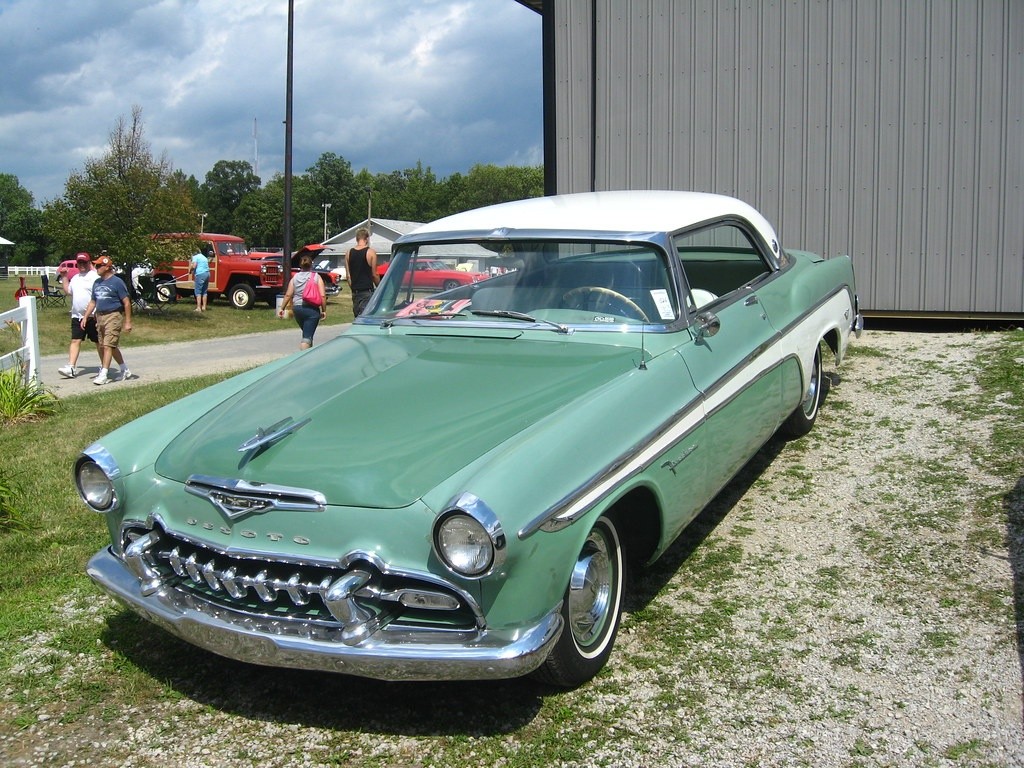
[114,368,133,382]
[58,366,77,378]
[93,374,108,385]
[95,366,111,375]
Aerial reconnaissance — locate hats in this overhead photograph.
[75,253,89,262]
[92,256,113,266]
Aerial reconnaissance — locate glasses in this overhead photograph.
[96,264,107,269]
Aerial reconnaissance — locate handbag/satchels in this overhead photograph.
[302,271,321,307]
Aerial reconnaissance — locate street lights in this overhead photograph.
[321,202,331,241]
[197,213,208,233]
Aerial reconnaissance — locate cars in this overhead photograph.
[375,259,489,293]
[54,259,117,285]
[132,233,344,311]
[68,189,865,693]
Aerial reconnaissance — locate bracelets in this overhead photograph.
[322,311,325,312]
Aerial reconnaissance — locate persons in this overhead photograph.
[485,266,517,277]
[344,229,381,318]
[189,247,210,312]
[58,253,101,378]
[80,256,131,385]
[277,256,327,350]
[111,262,155,310]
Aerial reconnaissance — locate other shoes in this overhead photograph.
[193,308,205,312]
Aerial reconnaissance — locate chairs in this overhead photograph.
[138,276,176,317]
[14,276,46,310]
[41,275,67,307]
[473,260,764,317]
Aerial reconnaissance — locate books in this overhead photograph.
[398,298,473,320]
